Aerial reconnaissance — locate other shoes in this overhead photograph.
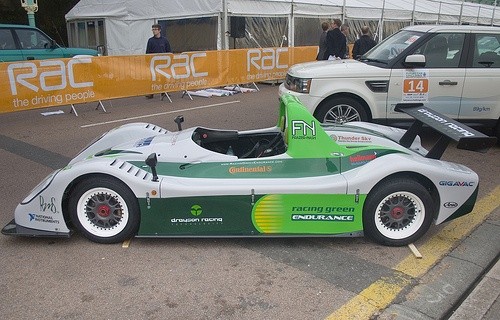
[161,93,170,97]
[145,94,153,98]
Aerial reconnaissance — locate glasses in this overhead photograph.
[152,28,159,31]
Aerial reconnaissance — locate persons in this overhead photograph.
[145,24,171,98]
[352,26,377,60]
[316,22,329,60]
[341,24,350,58]
[325,18,346,59]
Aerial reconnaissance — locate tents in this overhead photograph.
[65,0,500,83]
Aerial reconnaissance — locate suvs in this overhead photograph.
[277,22,500,134]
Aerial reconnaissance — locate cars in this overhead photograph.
[0,24,101,62]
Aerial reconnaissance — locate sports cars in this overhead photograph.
[0,93,500,248]
